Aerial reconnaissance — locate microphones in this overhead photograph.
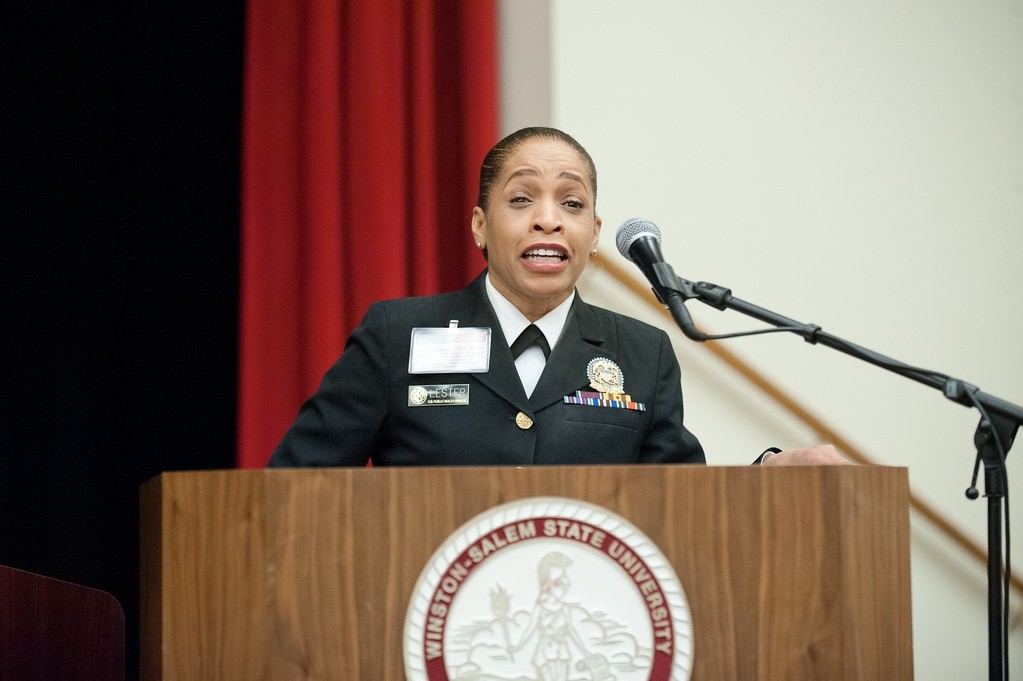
[615,217,700,340]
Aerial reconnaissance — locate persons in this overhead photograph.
[267,127,849,467]
